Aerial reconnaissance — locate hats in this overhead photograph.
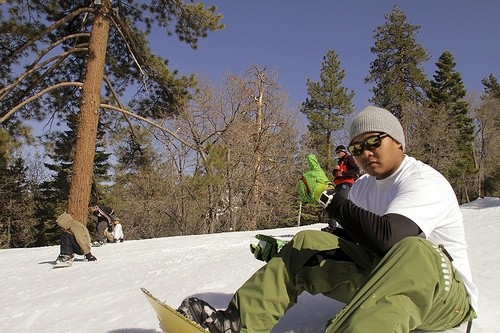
[349,106,405,153]
[335,145,346,151]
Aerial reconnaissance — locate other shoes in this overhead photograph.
[55,253,75,264]
[178,296,241,333]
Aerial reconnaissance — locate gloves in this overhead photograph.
[85,252,97,261]
[298,155,331,203]
[249,234,288,263]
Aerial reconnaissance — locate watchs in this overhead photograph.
[315,189,339,209]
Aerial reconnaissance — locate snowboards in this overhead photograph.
[91,238,107,247]
[141,287,211,333]
[53,254,75,267]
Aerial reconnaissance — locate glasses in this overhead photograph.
[346,134,389,156]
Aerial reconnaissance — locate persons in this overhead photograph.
[180,105,479,333]
[105,217,124,243]
[88,202,115,247]
[322,145,361,230]
[54,212,97,263]
